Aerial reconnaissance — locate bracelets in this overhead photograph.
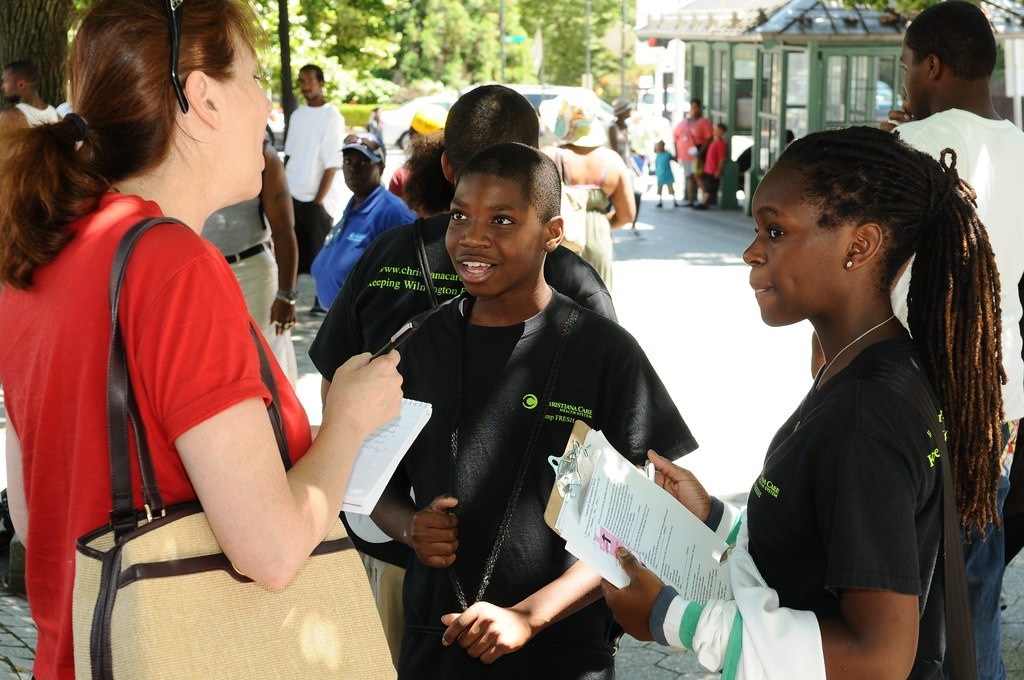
[277,290,299,305]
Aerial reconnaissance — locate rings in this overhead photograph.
[276,322,294,329]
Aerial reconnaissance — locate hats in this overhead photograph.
[337,133,387,162]
[410,104,448,136]
[612,98,631,116]
[557,114,607,148]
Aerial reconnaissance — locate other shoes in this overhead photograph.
[311,297,328,315]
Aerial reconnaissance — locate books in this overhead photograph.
[342,397,432,515]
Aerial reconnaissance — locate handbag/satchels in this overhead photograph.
[556,146,591,252]
[71,218,400,680]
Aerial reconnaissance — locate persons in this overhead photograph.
[0,61,57,125]
[813,0,1024,680]
[602,126,1006,680]
[309,84,618,413]
[366,141,699,680]
[285,63,451,316]
[539,86,637,293]
[201,136,299,391]
[0,0,403,680]
[608,99,645,234]
[649,99,727,210]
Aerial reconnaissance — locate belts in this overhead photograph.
[224,240,270,264]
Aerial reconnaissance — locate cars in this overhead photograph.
[376,85,470,151]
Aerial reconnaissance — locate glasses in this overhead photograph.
[342,135,384,162]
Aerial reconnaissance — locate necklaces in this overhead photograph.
[795,314,894,430]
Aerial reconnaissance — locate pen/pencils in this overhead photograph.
[369,321,420,363]
[646,463,655,484]
